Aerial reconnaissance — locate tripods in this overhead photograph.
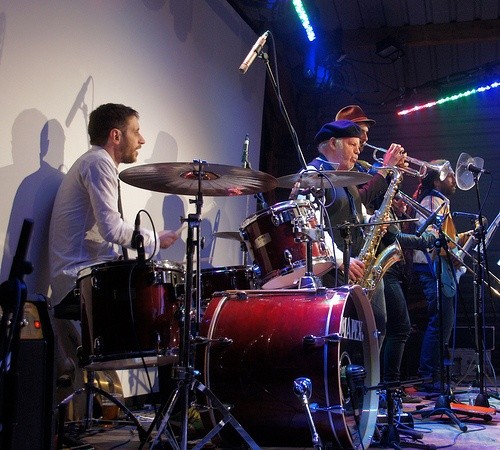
[146,172,264,450]
[370,173,500,450]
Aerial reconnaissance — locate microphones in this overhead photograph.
[238,31,268,74]
[466,163,491,174]
[242,136,249,169]
[289,175,303,199]
[415,201,445,237]
[131,214,141,249]
[453,212,479,218]
[345,364,368,427]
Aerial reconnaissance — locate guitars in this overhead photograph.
[438,226,479,299]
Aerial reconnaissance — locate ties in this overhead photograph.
[117,178,128,261]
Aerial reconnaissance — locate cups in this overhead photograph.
[101,393,122,427]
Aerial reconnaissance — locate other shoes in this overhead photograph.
[414,373,456,390]
[402,395,423,402]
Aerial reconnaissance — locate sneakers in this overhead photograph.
[167,406,204,436]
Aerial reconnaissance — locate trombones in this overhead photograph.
[357,159,500,297]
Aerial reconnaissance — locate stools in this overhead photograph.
[86,353,169,432]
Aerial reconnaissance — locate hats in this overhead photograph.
[335,105,376,126]
[314,120,363,146]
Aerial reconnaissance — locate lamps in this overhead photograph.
[375,36,402,59]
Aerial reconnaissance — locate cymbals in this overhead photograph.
[211,232,242,241]
[117,162,276,197]
[274,171,374,190]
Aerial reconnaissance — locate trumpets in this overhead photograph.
[362,140,451,182]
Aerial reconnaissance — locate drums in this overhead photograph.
[75,259,189,369]
[189,264,264,314]
[240,198,337,290]
[191,284,381,450]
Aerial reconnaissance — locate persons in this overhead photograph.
[289,119,387,354]
[48,104,207,436]
[335,104,486,402]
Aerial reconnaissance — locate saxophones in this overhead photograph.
[346,158,406,298]
